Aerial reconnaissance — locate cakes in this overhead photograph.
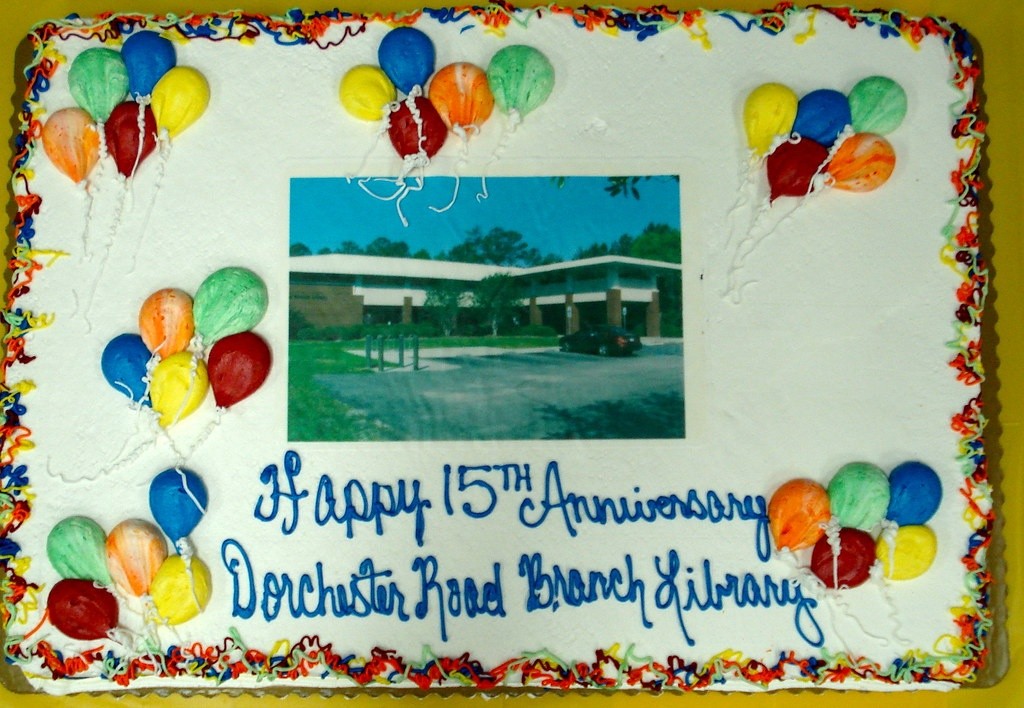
[1,1,1014,702]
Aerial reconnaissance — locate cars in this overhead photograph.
[559,323,643,357]
